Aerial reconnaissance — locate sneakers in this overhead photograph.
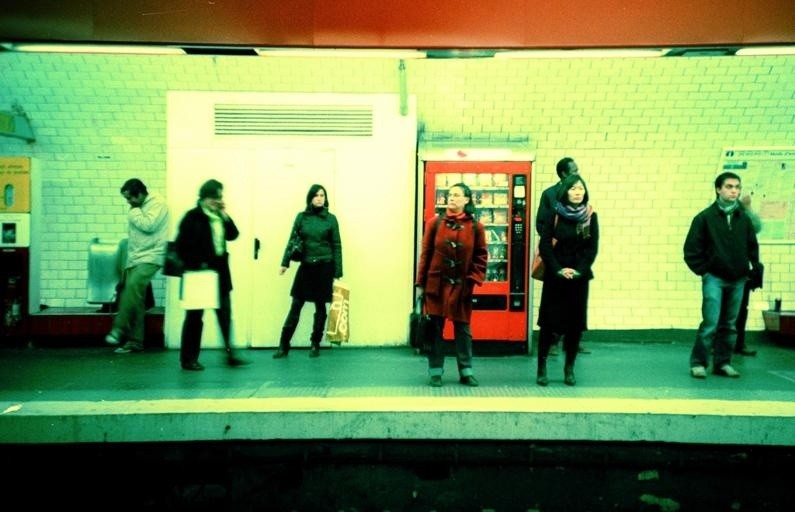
[691,365,707,378]
[105,333,144,353]
[717,365,738,378]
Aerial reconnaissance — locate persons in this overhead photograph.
[272,182,343,359]
[534,174,602,389]
[710,191,757,356]
[682,171,758,379]
[103,176,175,355]
[174,178,254,370]
[413,183,490,389]
[536,154,592,357]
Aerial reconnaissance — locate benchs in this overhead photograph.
[33,305,165,349]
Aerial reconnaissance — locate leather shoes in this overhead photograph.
[537,368,548,385]
[181,361,204,370]
[460,376,478,386]
[565,368,576,386]
[739,347,757,356]
[431,376,441,386]
[275,350,288,357]
[308,348,319,357]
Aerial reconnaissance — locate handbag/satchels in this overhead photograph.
[165,242,183,275]
[532,242,559,280]
[409,295,435,353]
[287,233,303,261]
